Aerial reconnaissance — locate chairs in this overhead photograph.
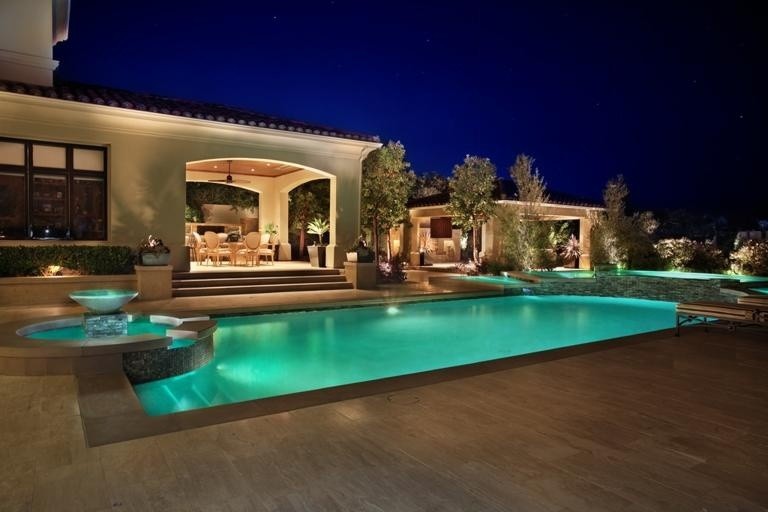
[184,231,279,267]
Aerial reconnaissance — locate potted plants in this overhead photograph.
[139,234,172,266]
[306,219,330,267]
[345,234,375,262]
[559,234,580,268]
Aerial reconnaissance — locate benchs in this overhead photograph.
[674,301,768,335]
[736,295,768,328]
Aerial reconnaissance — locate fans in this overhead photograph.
[208,160,251,183]
